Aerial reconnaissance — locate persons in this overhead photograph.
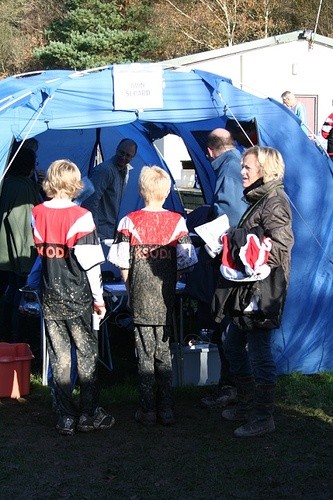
[32,159,116,436]
[107,163,198,428]
[1,137,138,343]
[321,97,333,163]
[194,128,295,440]
[281,91,307,137]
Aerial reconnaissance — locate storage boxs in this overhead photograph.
[0,342,35,398]
[172,342,222,386]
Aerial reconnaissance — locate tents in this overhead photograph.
[0,61,332,377]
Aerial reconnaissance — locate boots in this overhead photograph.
[234,382,276,436]
[222,375,255,421]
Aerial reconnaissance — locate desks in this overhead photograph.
[18,281,187,385]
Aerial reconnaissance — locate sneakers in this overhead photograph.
[202,385,238,408]
[134,409,175,425]
[57,407,115,435]
